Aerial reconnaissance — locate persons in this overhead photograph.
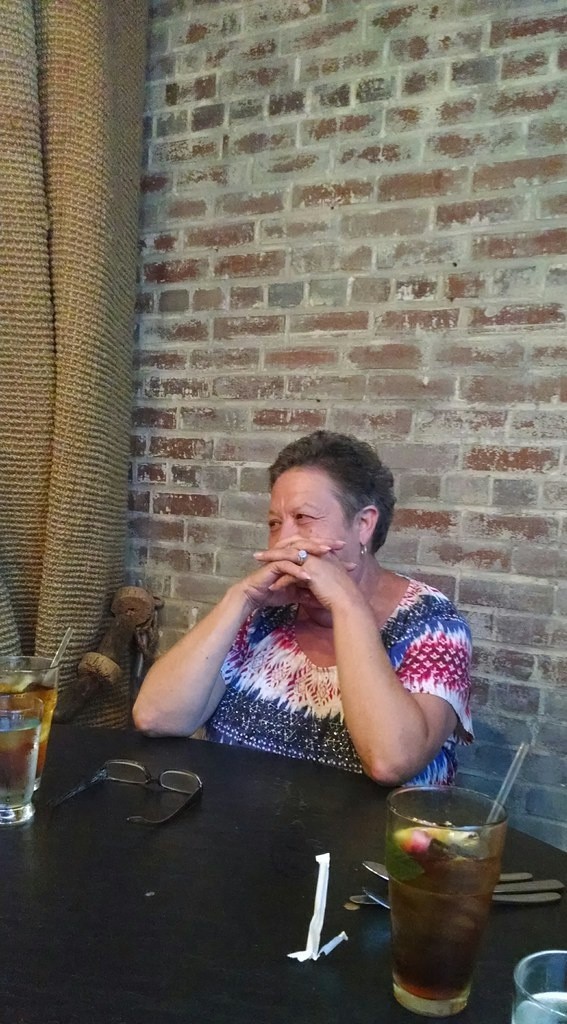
[132,429,475,788]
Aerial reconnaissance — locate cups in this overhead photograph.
[0,653,59,790]
[510,948,567,1024]
[0,694,45,826]
[383,785,509,1017]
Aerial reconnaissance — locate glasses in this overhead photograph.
[45,758,205,827]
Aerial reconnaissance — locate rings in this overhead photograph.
[299,549,306,566]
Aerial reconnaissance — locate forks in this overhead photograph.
[361,887,562,909]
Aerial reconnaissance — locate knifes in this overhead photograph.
[351,879,564,902]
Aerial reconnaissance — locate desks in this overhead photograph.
[0,728,566,1024]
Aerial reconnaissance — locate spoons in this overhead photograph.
[360,860,533,882]
